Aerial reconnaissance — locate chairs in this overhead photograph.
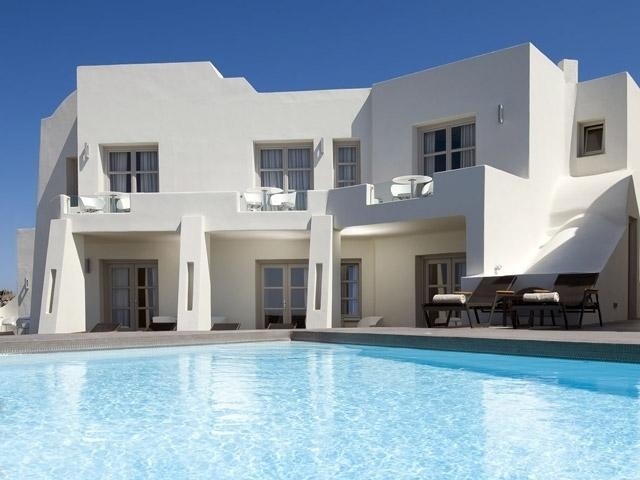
[79,196,104,213]
[358,316,383,327]
[496,271,603,331]
[117,197,130,212]
[211,321,241,330]
[420,275,517,328]
[267,322,297,329]
[143,322,176,331]
[242,188,297,211]
[390,181,433,200]
[88,322,121,332]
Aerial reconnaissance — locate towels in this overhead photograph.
[523,291,560,302]
[432,294,466,304]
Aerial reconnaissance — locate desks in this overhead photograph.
[251,187,284,210]
[393,175,432,199]
[489,289,514,325]
[95,192,117,213]
